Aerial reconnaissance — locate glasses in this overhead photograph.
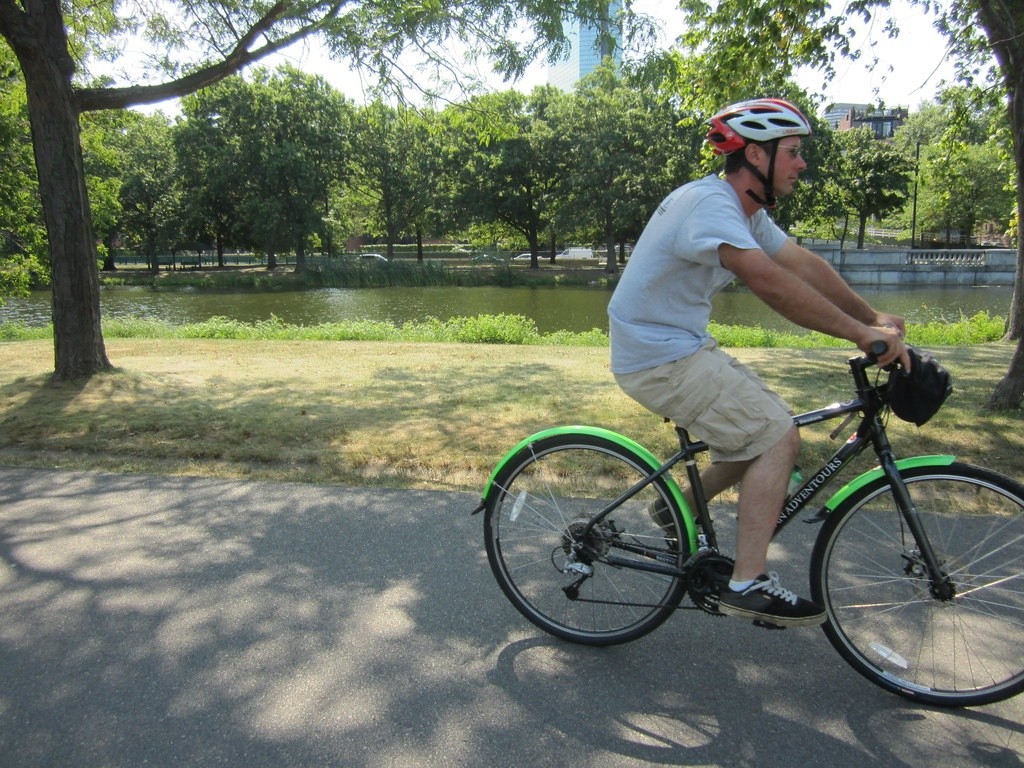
[761,144,808,156]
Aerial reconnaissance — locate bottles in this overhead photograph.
[787,469,802,499]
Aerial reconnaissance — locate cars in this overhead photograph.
[472,254,505,263]
[511,254,543,261]
[359,254,389,262]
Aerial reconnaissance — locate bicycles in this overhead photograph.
[471,325,1024,708]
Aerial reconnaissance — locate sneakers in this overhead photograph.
[719,570,827,627]
[647,496,717,550]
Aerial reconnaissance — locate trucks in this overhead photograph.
[556,247,594,259]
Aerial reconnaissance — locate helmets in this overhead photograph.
[702,98,812,155]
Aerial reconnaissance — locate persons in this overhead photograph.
[608,99,913,628]
